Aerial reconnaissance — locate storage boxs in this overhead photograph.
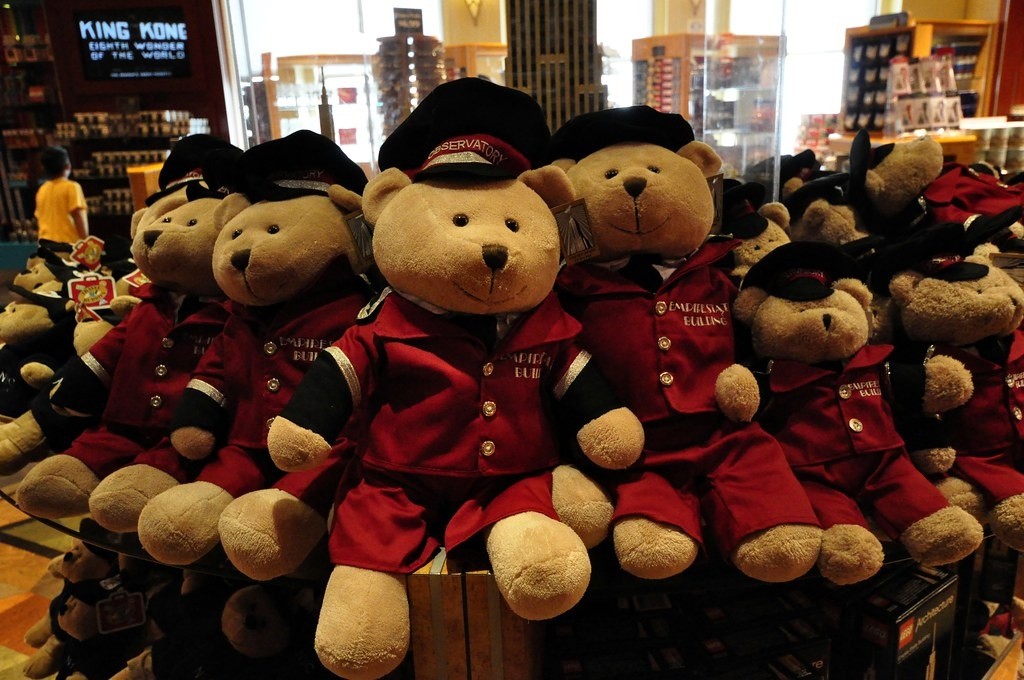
[541,528,1020,680]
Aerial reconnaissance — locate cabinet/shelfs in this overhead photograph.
[266,10,1024,176]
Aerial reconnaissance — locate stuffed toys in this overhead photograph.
[0,76,1024,680]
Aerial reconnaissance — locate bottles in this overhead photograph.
[2,128,46,151]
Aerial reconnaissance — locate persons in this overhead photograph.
[34,144,88,246]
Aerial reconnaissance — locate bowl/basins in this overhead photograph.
[895,118,1024,172]
